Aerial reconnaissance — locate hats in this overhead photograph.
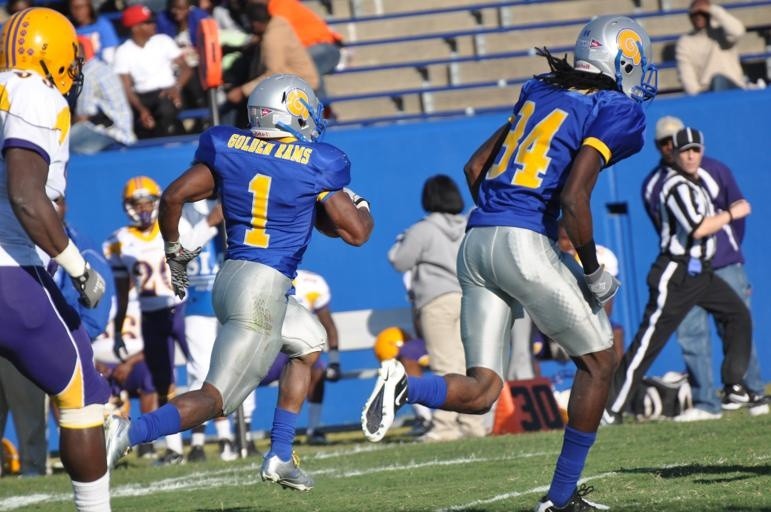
[673,126,703,152]
[123,4,154,27]
[655,118,681,141]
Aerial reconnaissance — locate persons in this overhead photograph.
[673,4,766,95]
[100,71,373,491]
[1,7,114,510]
[361,14,658,512]
[2,3,343,157]
[0,116,754,474]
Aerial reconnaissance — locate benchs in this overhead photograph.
[230,308,418,459]
[320,0,768,124]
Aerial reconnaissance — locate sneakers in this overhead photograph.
[598,409,624,428]
[537,484,609,511]
[721,384,768,411]
[261,453,313,491]
[675,409,721,422]
[104,415,129,470]
[362,359,406,441]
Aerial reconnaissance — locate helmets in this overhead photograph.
[0,7,83,101]
[574,15,657,107]
[125,174,161,226]
[248,73,327,142]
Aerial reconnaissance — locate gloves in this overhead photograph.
[582,264,621,305]
[166,247,200,297]
[70,263,106,308]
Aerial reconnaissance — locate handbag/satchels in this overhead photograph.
[636,377,691,419]
